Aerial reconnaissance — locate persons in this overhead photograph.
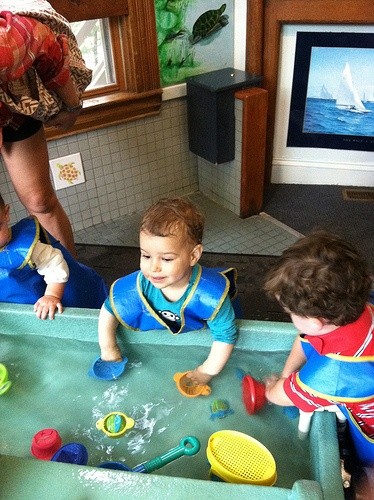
[97,194,242,385]
[0,194,108,319]
[264,229,374,466]
[0,9,83,259]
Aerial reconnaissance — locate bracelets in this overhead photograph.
[66,102,85,113]
